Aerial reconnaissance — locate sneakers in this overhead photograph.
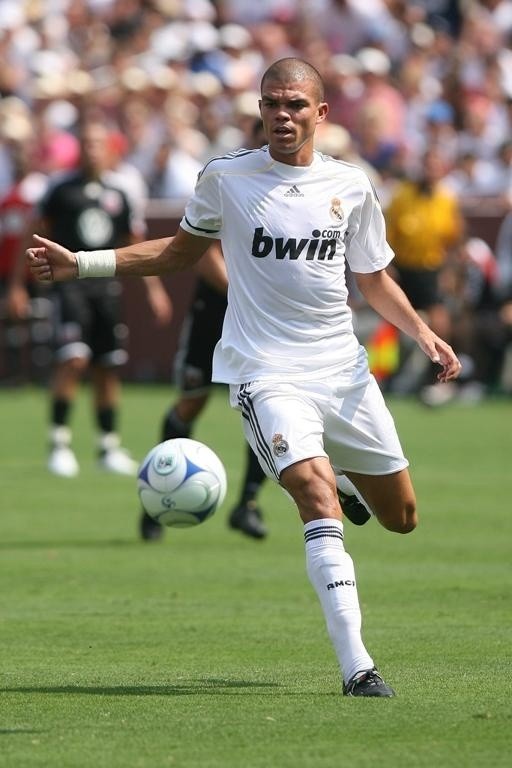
[228,502,267,538]
[342,666,396,698]
[140,510,162,541]
[336,487,371,526]
[45,427,140,477]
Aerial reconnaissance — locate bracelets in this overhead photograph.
[74,249,117,280]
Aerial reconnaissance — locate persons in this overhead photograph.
[12,121,173,478]
[24,58,463,697]
[0,0,512,412]
[142,119,273,545]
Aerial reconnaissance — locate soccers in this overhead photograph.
[133,437,229,530]
